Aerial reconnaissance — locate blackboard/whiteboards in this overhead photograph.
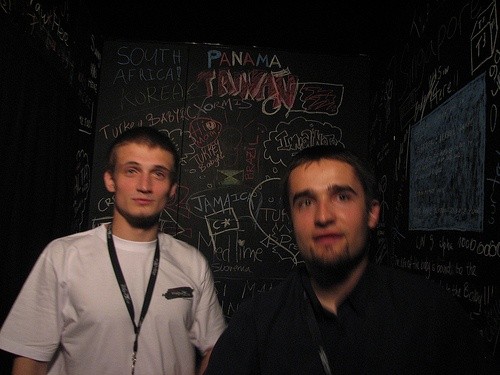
[367,0,500,359]
[88,43,365,331]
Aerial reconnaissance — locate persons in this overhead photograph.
[0,127,229,375]
[209,145,495,374]
